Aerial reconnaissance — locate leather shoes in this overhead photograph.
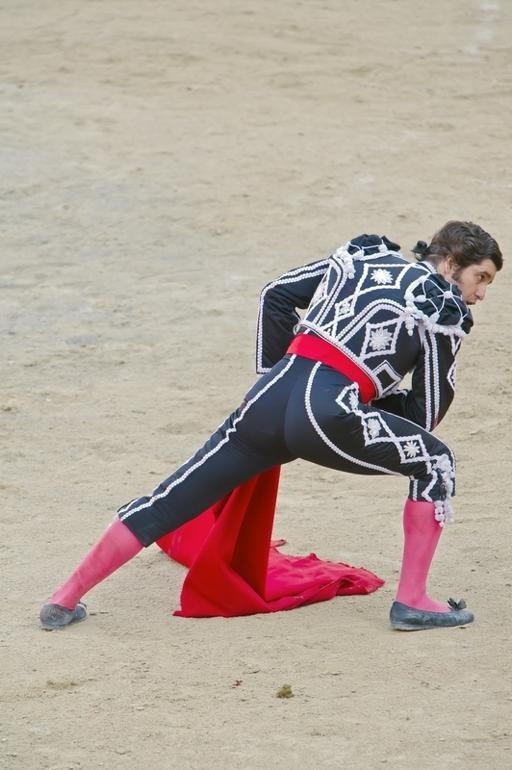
[38,601,89,631]
[391,598,476,631]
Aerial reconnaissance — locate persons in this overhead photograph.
[39,219,504,633]
